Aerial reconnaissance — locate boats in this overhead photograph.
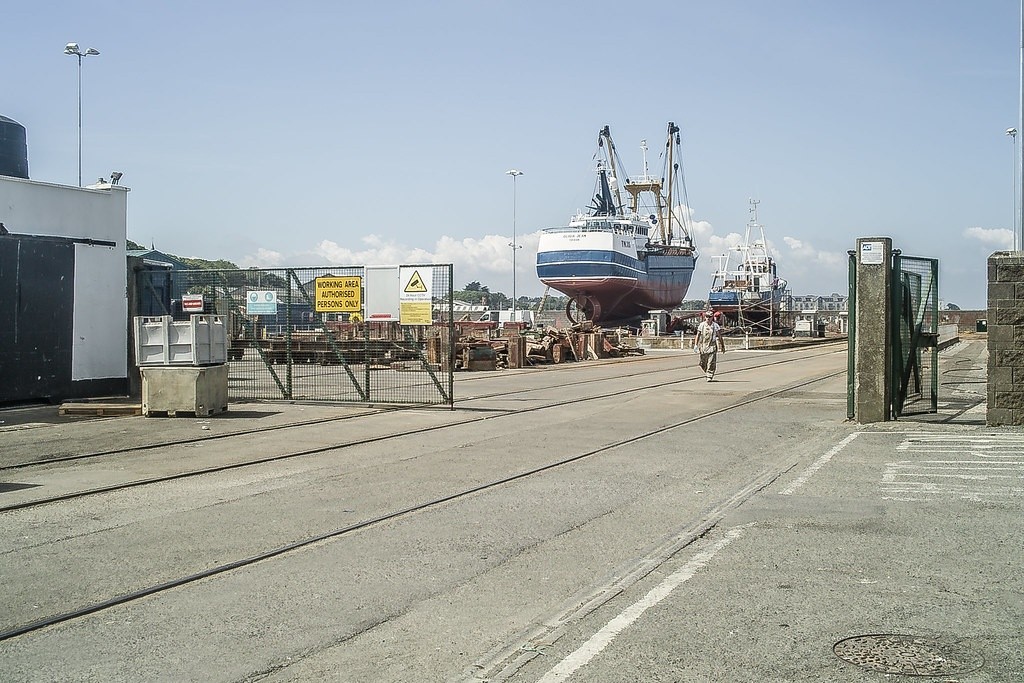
[536,121,700,320]
[707,196,787,329]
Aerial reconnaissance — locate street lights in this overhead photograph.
[1006,127,1017,252]
[505,168,526,323]
[64,42,101,185]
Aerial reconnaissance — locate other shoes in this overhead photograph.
[707,378,711,381]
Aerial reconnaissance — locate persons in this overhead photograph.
[694,311,725,382]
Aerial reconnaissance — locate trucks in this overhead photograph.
[476,310,535,322]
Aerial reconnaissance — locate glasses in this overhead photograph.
[705,316,712,318]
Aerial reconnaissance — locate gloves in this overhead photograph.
[693,346,698,353]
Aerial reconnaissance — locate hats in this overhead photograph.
[704,311,714,316]
